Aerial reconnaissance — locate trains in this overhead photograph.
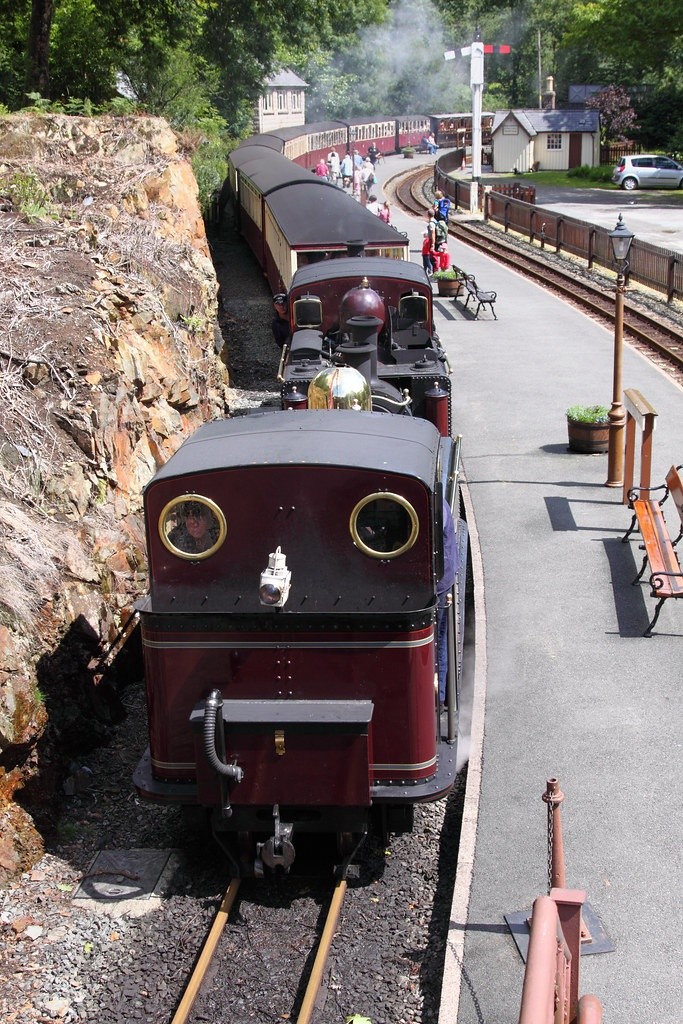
[131,110,495,876]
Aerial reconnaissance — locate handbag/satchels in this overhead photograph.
[369,173,373,181]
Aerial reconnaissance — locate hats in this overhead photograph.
[366,157,371,162]
[421,231,428,235]
[273,294,287,304]
[439,243,448,248]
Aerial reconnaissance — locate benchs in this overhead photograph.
[453,265,499,321]
[621,464,683,639]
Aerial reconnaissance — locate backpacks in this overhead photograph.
[436,198,450,219]
[429,220,448,244]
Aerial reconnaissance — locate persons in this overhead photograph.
[421,190,451,283]
[272,294,290,350]
[316,141,390,224]
[167,501,219,554]
[421,121,453,154]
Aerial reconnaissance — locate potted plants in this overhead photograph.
[432,271,464,297]
[566,404,610,452]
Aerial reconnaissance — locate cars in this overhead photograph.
[611,155,683,190]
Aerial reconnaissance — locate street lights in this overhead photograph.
[607,212,636,492]
[347,129,358,196]
